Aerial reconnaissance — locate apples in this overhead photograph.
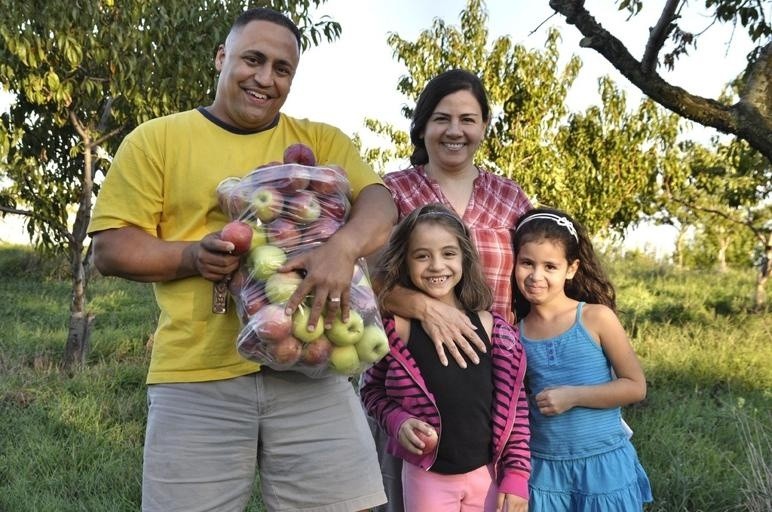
[216,144,389,380]
[413,425,439,455]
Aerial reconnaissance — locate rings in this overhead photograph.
[328,298,341,302]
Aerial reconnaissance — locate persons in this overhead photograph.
[365,66,535,512]
[85,7,402,512]
[357,202,534,512]
[515,205,650,512]
